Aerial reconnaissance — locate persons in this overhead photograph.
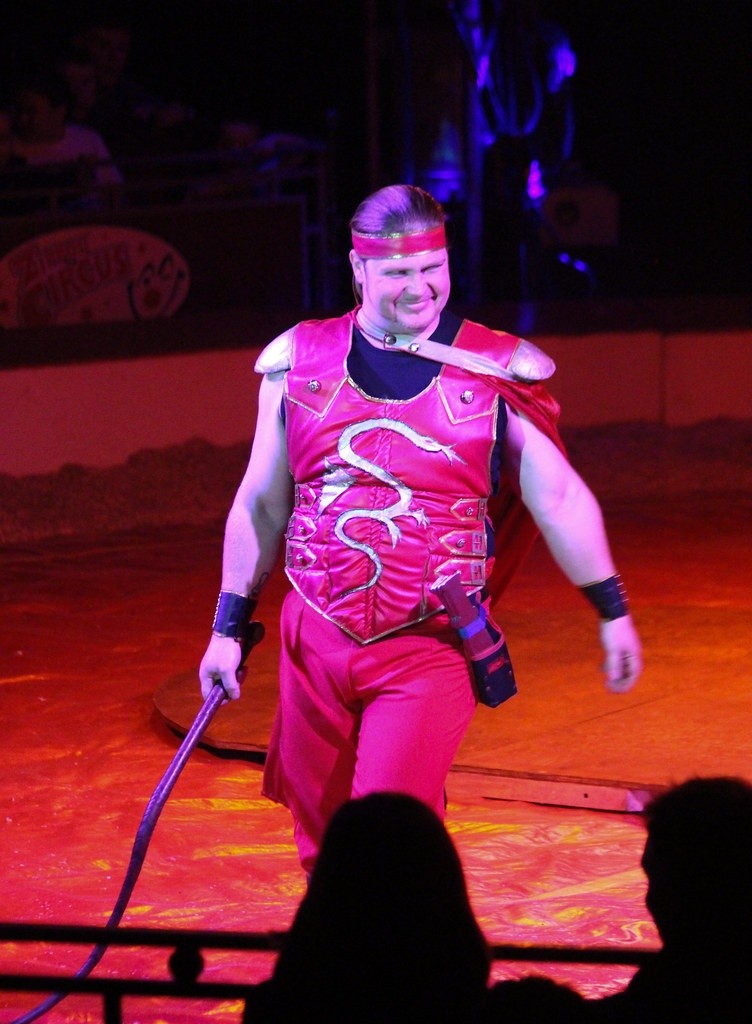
[494,774,752,1023]
[0,22,302,221]
[241,794,494,1023]
[198,181,643,894]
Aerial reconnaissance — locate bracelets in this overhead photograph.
[211,590,257,641]
[572,570,629,622]
